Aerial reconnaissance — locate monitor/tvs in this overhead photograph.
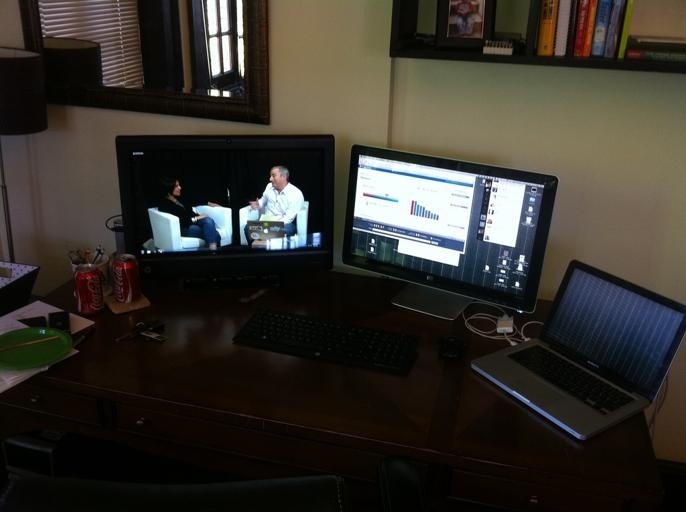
[113,133,336,290]
[341,144,559,320]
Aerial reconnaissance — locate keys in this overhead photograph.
[115,323,145,341]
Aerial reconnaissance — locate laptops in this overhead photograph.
[471,258,686,438]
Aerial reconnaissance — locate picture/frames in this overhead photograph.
[434,0,497,48]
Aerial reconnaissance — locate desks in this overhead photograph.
[1,272,660,512]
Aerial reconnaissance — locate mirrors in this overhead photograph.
[18,0,271,126]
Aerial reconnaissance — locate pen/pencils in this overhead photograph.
[68,244,106,265]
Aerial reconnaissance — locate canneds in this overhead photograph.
[112,253,140,304]
[73,264,105,314]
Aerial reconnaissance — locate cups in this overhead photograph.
[113,218,127,255]
[72,253,110,293]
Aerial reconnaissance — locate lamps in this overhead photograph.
[42,37,102,87]
[0,46,48,262]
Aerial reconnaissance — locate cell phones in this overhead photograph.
[49,311,70,335]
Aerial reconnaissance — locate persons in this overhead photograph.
[244,165,305,244]
[157,176,222,252]
[454,1,478,37]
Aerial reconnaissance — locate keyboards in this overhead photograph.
[230,307,420,375]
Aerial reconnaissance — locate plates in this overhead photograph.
[74,286,114,297]
[0,326,71,371]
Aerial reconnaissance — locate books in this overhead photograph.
[525,1,636,61]
[624,36,686,65]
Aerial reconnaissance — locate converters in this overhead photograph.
[498,314,512,334]
